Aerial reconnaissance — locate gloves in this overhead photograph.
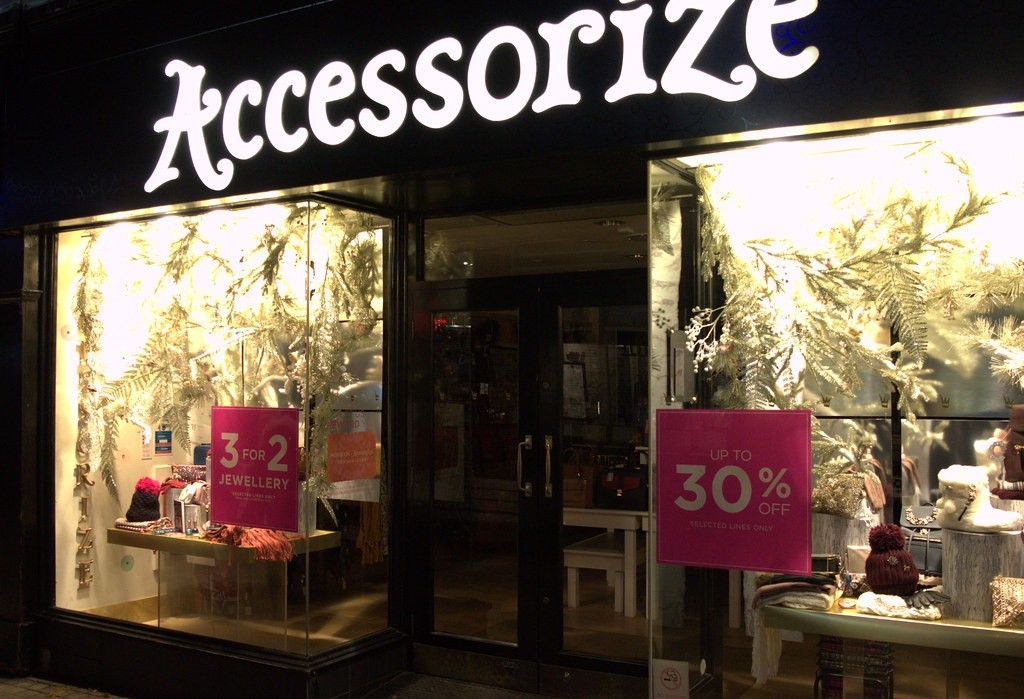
[907,589,952,608]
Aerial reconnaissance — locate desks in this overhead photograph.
[563,504,647,616]
[105,523,341,622]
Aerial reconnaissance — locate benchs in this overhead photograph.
[566,535,646,618]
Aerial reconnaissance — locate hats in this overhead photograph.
[864,523,919,596]
[126,477,161,522]
